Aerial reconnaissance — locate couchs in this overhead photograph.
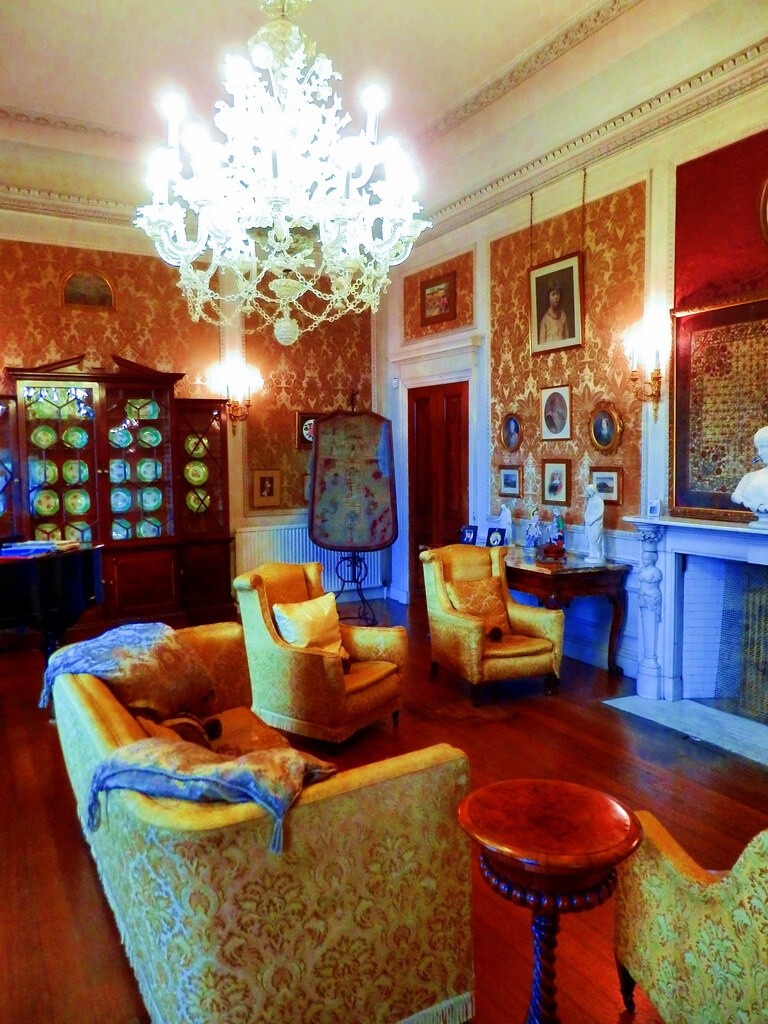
[419,547,566,707]
[235,563,410,741]
[48,621,471,1023]
[613,811,768,1024]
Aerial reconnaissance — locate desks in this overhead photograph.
[504,546,633,697]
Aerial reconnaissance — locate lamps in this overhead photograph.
[628,346,663,424]
[133,0,430,343]
[210,350,262,422]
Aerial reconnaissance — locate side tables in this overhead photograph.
[457,781,641,1024]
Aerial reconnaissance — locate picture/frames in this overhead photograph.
[251,469,286,508]
[486,527,507,547]
[500,415,524,450]
[499,465,526,498]
[586,400,623,453]
[419,271,459,326]
[541,458,573,506]
[460,526,478,547]
[294,411,327,447]
[539,385,575,441]
[528,252,587,354]
[587,466,626,505]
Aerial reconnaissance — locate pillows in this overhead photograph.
[271,593,348,662]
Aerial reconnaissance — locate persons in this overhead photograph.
[539,277,569,345]
[731,426,768,513]
[492,504,513,545]
[583,484,605,558]
[638,552,662,658]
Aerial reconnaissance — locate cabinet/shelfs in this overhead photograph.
[0,352,231,634]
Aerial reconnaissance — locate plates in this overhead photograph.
[30,424,210,542]
